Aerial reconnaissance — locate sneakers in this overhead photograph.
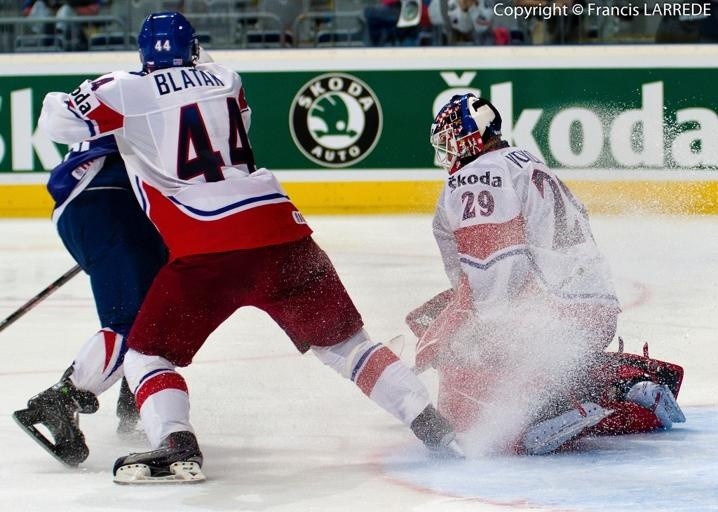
[410,404,452,451]
[114,433,203,470]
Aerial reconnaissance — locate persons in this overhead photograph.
[13,117,154,469]
[412,92,678,449]
[21,1,100,43]
[42,13,476,482]
[374,0,718,45]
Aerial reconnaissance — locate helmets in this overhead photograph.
[428,94,502,170]
[136,12,200,69]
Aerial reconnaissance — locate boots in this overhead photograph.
[26,361,99,463]
[111,375,150,450]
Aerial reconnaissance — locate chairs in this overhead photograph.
[0,1,380,52]
